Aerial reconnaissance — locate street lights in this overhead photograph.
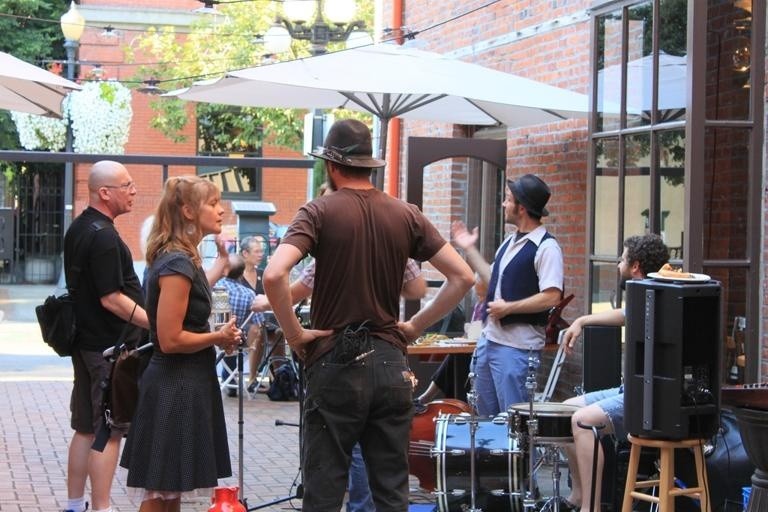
[262,0,379,153]
[58,0,89,288]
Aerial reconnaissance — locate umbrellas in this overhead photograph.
[162,39,594,195]
[0,47,83,122]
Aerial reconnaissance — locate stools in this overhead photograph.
[619,433,713,512]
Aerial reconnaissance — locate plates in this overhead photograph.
[414,338,476,346]
[646,272,712,283]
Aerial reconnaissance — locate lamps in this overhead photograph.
[78,1,433,103]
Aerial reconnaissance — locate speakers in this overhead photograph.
[621,278,722,442]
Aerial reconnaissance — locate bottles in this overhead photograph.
[209,286,233,335]
[207,486,248,512]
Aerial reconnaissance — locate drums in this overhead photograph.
[434,412,523,512]
[407,399,478,491]
[508,402,583,444]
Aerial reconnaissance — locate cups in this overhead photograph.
[467,320,483,340]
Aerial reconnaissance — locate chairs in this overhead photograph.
[531,326,571,403]
[208,292,309,400]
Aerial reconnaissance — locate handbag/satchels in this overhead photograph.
[35,291,84,358]
[86,340,146,452]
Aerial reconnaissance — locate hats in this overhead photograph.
[507,173,552,217]
[308,118,388,169]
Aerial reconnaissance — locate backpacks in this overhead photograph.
[267,363,298,402]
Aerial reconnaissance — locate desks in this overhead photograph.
[407,336,478,398]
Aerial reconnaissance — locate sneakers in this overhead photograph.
[62,500,89,512]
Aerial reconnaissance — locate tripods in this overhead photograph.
[538,446,578,512]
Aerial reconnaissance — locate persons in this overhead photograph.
[250,256,428,313]
[64,161,150,512]
[449,173,562,422]
[563,234,670,510]
[212,254,269,395]
[121,175,243,512]
[261,118,475,512]
[222,237,285,397]
